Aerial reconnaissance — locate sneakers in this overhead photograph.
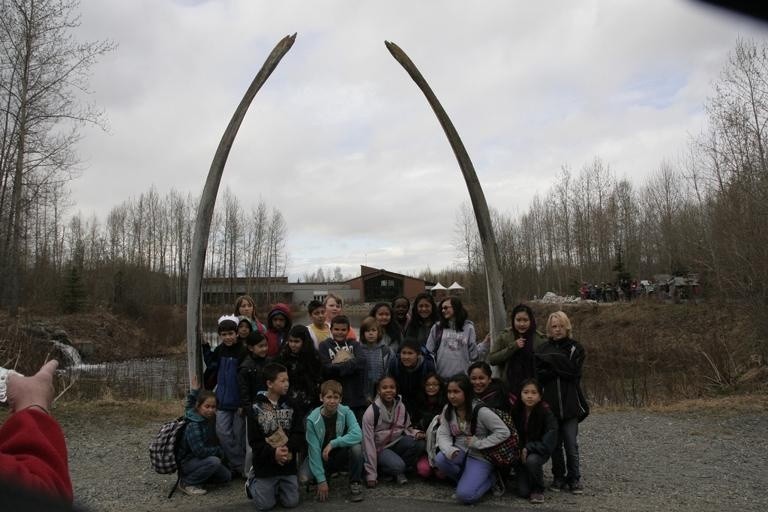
[492,470,506,498]
[549,480,566,492]
[176,479,207,498]
[347,481,363,503]
[396,473,408,485]
[244,465,256,501]
[531,492,545,504]
[570,479,584,495]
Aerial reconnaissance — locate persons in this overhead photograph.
[0,359,73,512]
[578,278,669,303]
[176,293,589,510]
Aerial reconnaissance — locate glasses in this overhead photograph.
[441,305,452,311]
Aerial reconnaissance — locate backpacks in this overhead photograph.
[149,415,188,476]
[470,402,518,464]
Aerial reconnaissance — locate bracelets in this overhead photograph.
[16,404,49,414]
